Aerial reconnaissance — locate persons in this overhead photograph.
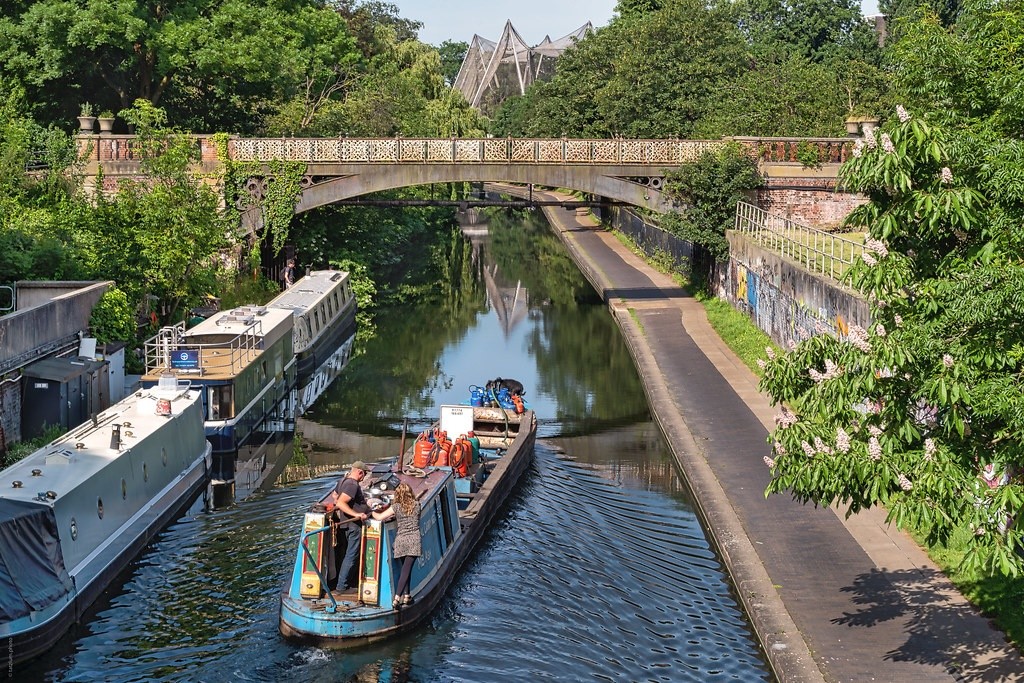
[284,260,295,290]
[369,484,422,611]
[331,461,370,595]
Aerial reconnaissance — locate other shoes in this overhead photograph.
[335,587,358,594]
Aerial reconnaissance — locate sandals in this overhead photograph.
[403,596,414,605]
[392,599,401,610]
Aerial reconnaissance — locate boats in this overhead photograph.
[263,267,360,381]
[2,374,215,670]
[209,357,302,486]
[298,332,358,415]
[276,376,539,648]
[135,303,299,454]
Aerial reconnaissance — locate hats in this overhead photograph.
[352,461,370,470]
